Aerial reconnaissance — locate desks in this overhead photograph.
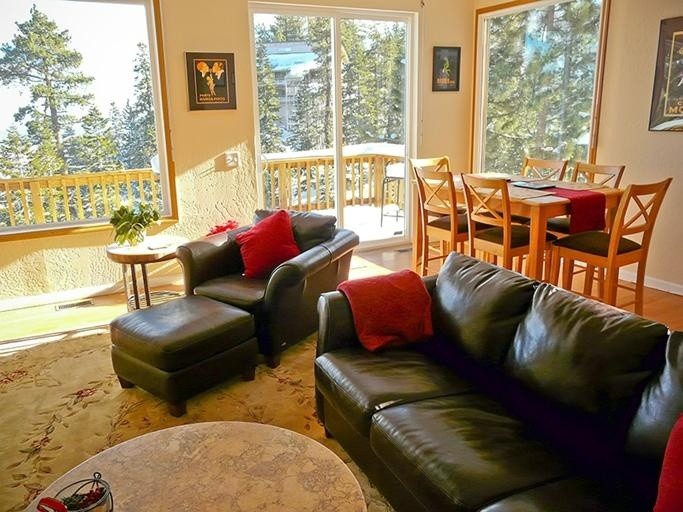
[106,235,189,309]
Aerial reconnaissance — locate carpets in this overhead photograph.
[0,331,400,512]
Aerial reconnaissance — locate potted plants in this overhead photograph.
[108,203,160,244]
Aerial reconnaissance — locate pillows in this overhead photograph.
[233,209,301,279]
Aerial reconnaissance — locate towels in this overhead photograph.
[335,268,435,352]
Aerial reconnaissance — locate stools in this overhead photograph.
[109,293,261,418]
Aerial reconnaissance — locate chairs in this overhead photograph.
[175,207,360,369]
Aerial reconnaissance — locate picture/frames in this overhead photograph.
[185,51,236,111]
[648,16,683,132]
[432,45,462,92]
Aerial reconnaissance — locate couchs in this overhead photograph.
[314,249,683,512]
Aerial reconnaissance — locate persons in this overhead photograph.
[206,61,227,97]
[196,61,213,94]
